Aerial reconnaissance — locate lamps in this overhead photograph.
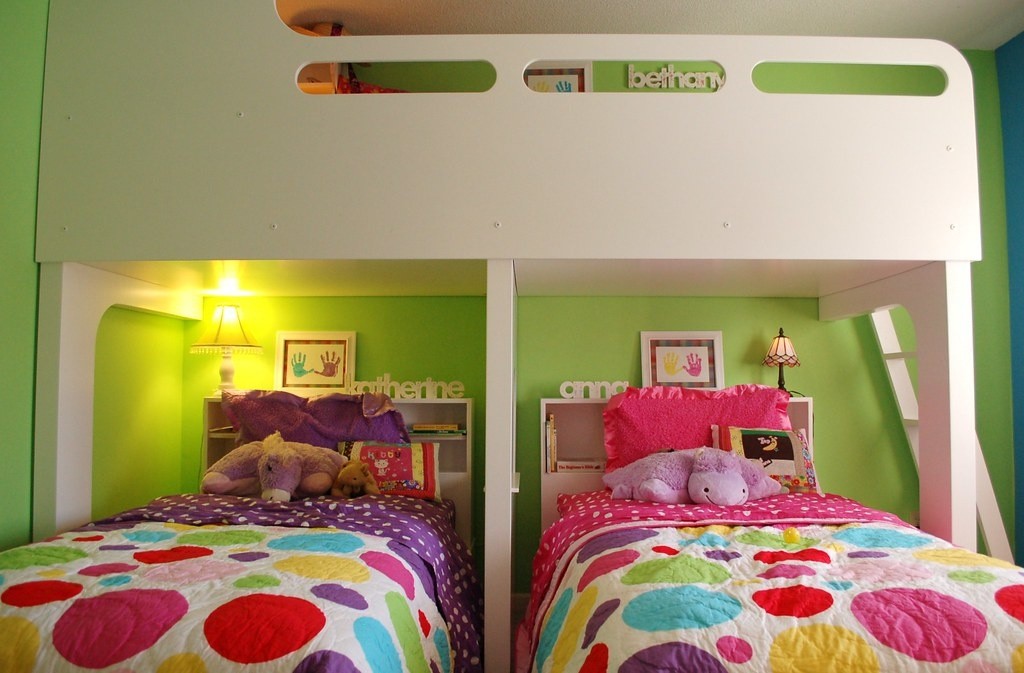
[761,327,802,398]
[186,303,264,396]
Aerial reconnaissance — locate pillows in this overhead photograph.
[334,440,442,504]
[709,423,825,499]
[219,389,410,450]
[603,382,792,497]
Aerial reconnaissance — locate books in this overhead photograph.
[545,413,607,472]
[405,421,466,437]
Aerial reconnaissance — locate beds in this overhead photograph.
[0,391,482,673]
[514,394,1023,673]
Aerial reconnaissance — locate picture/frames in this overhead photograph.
[522,60,593,91]
[639,329,725,393]
[275,330,357,395]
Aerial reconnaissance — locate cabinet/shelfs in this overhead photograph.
[202,396,474,550]
[541,396,813,534]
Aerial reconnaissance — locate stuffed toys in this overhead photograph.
[201,433,348,503]
[335,461,372,501]
[601,444,781,507]
